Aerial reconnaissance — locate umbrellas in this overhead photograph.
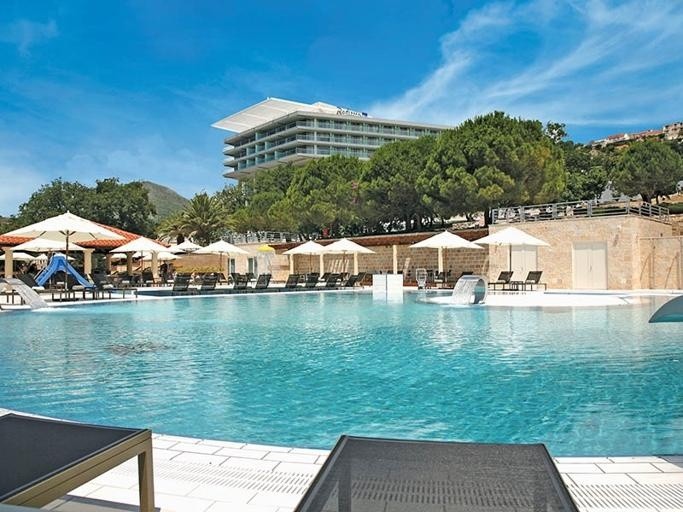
[321,238,376,280]
[408,231,486,286]
[472,226,550,288]
[282,241,327,276]
[0,210,252,298]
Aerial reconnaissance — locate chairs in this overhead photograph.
[0,412,154,511]
[294,435,578,511]
